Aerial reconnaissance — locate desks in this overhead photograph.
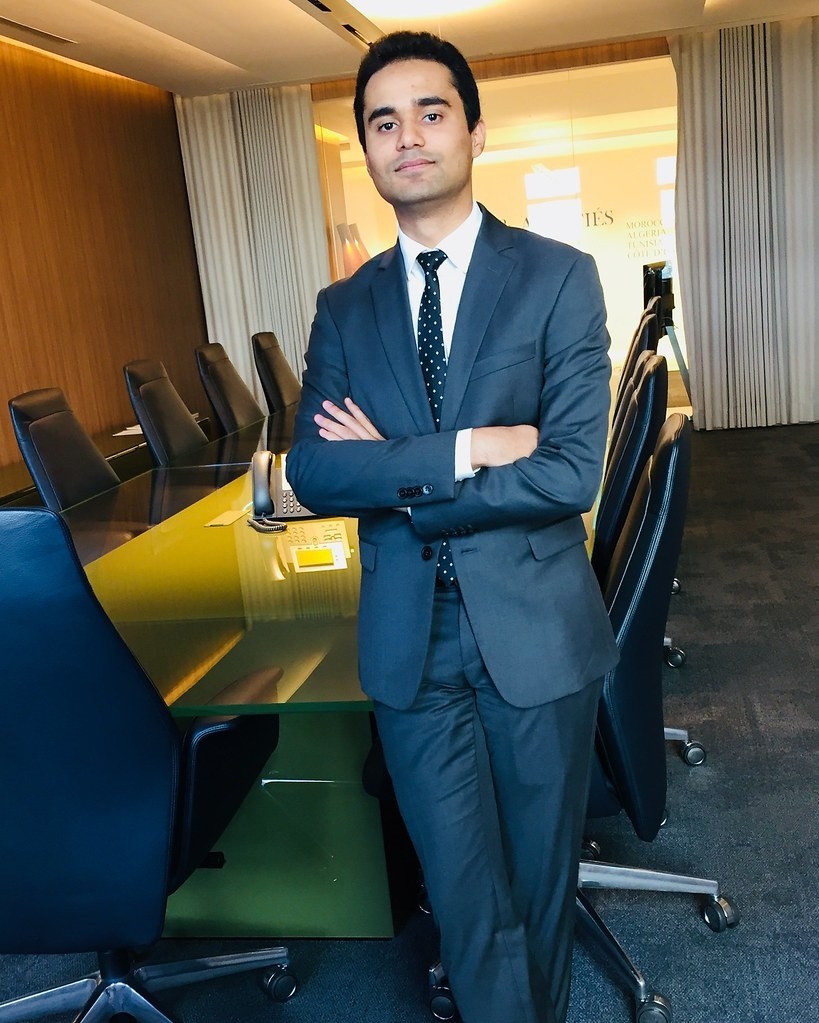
[78,435,385,966]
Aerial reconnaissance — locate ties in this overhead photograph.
[415,247,448,434]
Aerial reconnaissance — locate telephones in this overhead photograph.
[251,450,322,523]
[270,520,352,581]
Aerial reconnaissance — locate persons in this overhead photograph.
[284,30,623,1023]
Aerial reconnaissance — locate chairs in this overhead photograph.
[587,298,674,577]
[194,340,267,435]
[424,408,738,1019]
[121,359,212,464]
[251,327,305,411]
[0,508,303,1023]
[11,388,126,519]
